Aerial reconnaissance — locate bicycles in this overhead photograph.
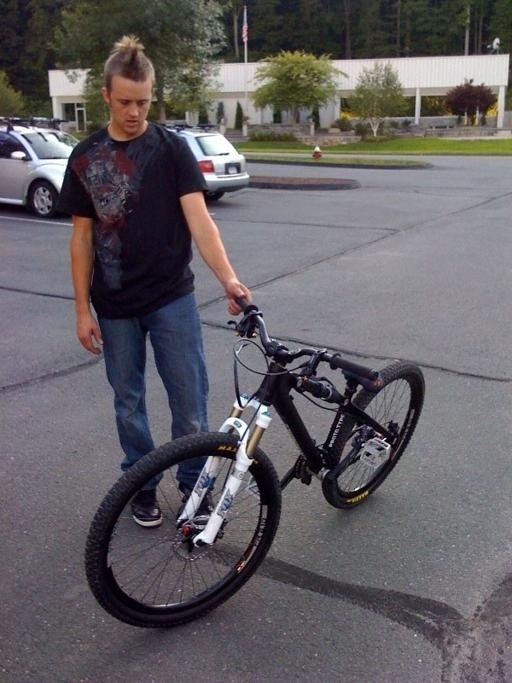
[84,287,427,633]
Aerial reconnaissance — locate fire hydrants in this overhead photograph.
[311,144,324,159]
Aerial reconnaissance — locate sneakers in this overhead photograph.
[131,488,162,527]
[178,484,214,515]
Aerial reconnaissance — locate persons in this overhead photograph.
[56,30,253,532]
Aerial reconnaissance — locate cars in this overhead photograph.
[1,113,84,216]
[159,120,251,200]
